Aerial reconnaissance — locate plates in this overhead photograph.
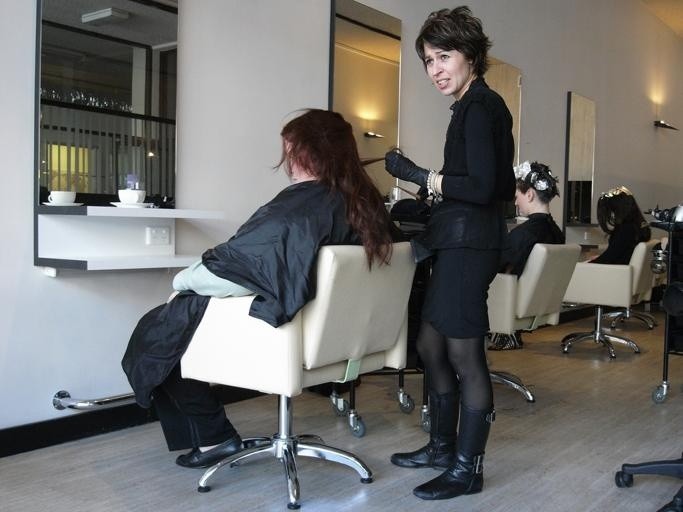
[110,202,158,209]
[42,201,85,208]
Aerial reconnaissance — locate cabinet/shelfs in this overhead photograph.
[33,205,225,272]
[649,218,682,405]
[562,223,608,249]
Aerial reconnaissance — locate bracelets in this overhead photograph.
[426,168,443,206]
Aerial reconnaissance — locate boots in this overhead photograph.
[412,403,495,499]
[390,390,459,470]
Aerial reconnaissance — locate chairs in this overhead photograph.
[561,238,662,361]
[593,234,668,332]
[166,241,417,508]
[454,243,581,400]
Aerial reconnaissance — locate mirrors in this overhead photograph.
[564,91,595,222]
[481,55,521,171]
[330,0,402,204]
[34,0,178,209]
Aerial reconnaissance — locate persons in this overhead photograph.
[487,162,563,280]
[122,108,406,468]
[588,185,650,264]
[385,6,513,501]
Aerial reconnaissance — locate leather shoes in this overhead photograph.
[176,435,244,470]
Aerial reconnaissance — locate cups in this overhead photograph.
[46,190,76,204]
[117,188,148,203]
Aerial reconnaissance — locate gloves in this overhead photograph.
[415,187,428,201]
[383,152,429,188]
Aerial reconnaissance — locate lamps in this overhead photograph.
[81,8,125,28]
